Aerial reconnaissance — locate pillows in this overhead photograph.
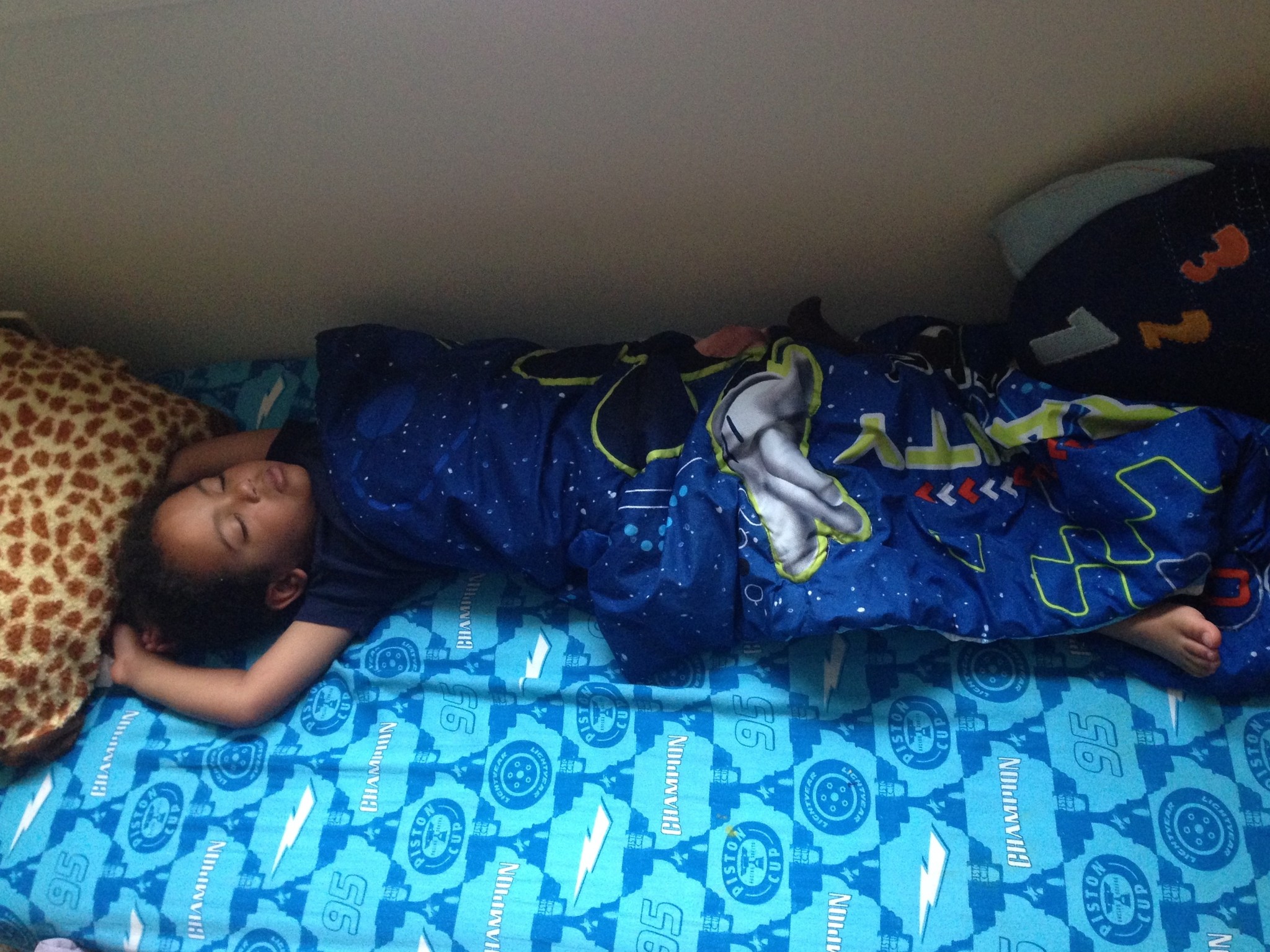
[0,311,245,771]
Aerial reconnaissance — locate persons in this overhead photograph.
[105,329,1223,728]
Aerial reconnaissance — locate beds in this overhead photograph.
[1,353,1270,952]
